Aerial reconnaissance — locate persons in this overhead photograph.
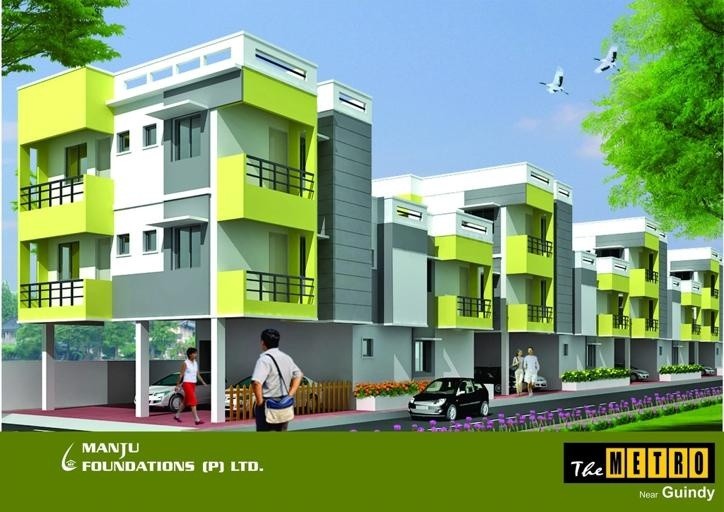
[522,345,540,396]
[251,328,303,432]
[512,348,524,397]
[173,347,208,425]
